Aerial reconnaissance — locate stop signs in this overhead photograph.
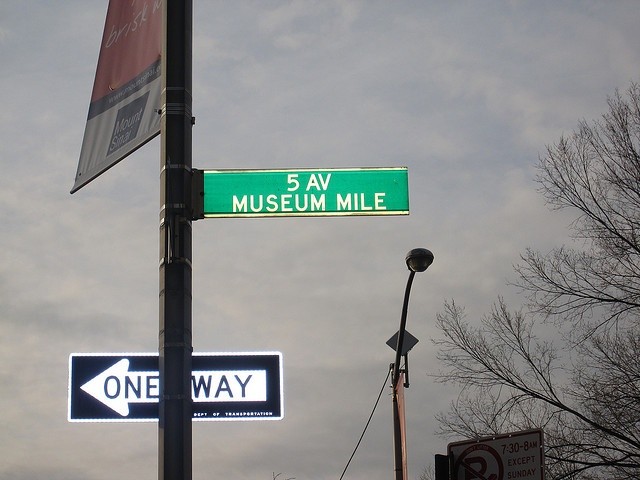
[447,429,544,480]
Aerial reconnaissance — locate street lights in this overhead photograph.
[391,248,433,480]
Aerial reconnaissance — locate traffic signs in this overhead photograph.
[67,352,284,422]
